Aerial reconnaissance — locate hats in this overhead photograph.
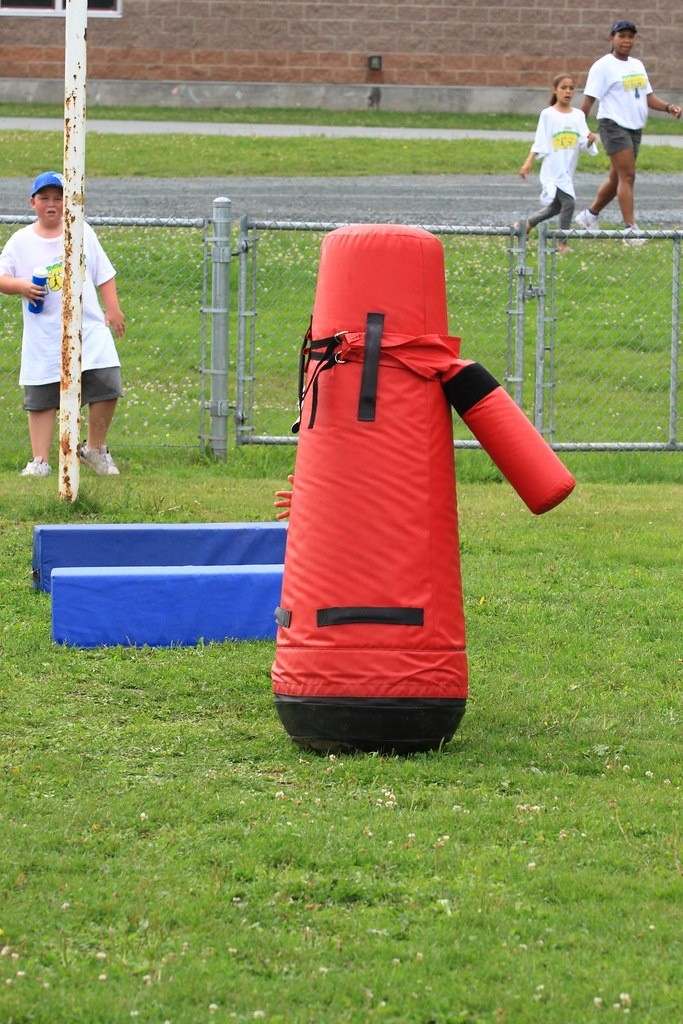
[29,170,65,200]
[612,18,638,35]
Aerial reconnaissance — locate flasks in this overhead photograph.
[28,265,50,313]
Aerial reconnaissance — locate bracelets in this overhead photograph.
[665,103,672,114]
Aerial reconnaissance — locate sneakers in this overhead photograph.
[573,209,603,238]
[78,439,122,479]
[622,224,650,246]
[20,457,53,480]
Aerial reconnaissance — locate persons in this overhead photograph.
[0,170,126,478]
[512,71,599,255]
[579,19,682,245]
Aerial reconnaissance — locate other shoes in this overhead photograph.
[513,221,530,241]
[556,243,575,255]
